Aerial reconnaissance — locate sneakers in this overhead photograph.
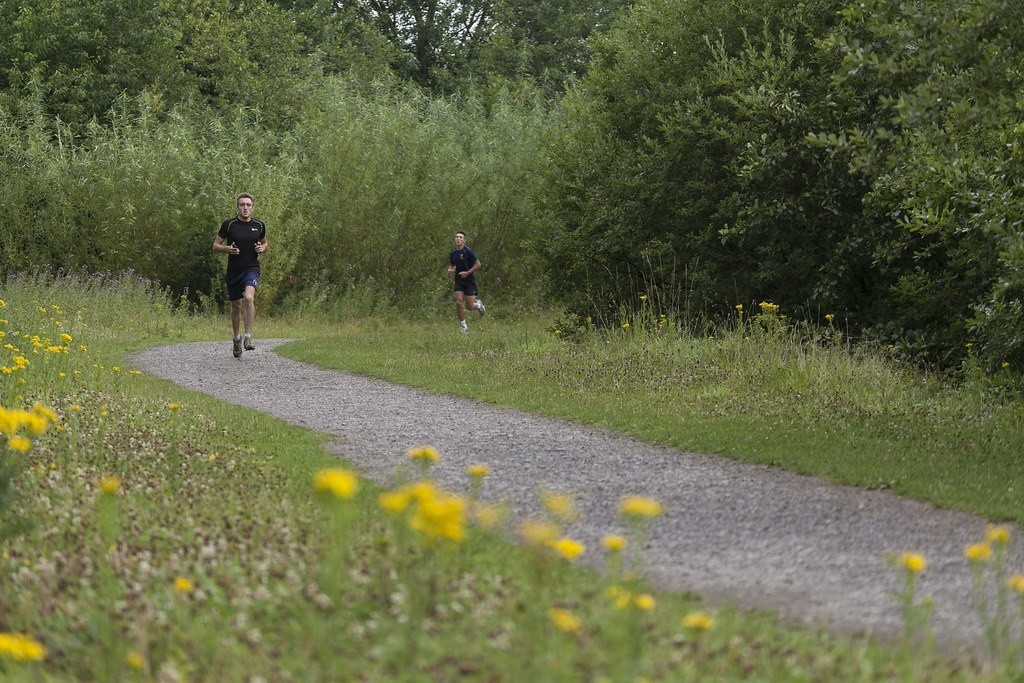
[244,336,255,350]
[476,299,485,316]
[459,325,468,335]
[232,335,242,357]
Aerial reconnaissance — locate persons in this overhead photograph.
[448,231,486,333]
[213,192,268,358]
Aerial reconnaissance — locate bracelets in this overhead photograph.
[468,271,470,275]
